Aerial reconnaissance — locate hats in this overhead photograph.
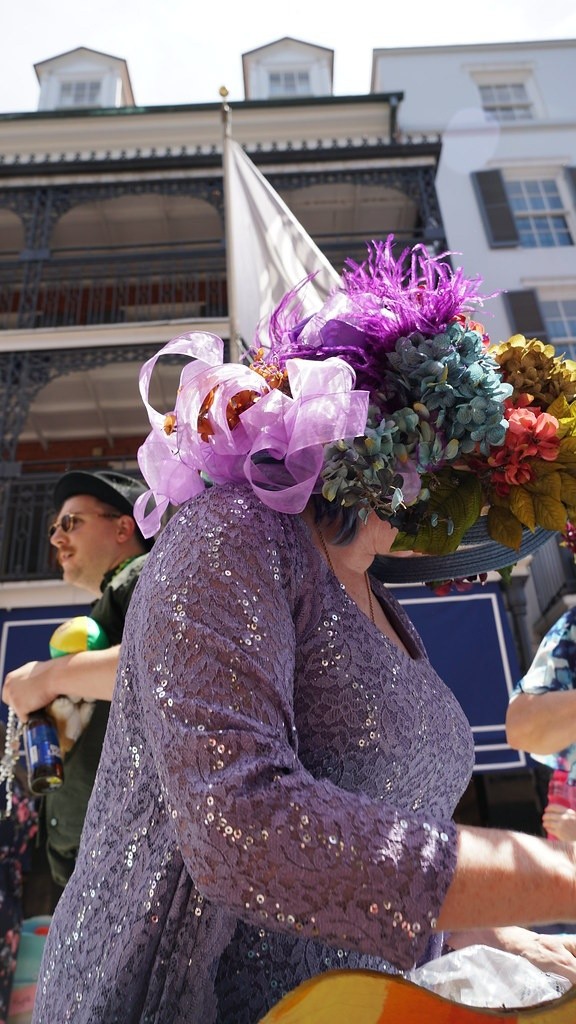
[54,471,159,551]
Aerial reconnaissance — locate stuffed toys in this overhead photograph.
[49,617,108,752]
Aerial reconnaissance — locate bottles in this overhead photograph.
[546,770,570,842]
[23,708,64,794]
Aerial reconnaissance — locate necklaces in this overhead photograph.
[317,523,375,623]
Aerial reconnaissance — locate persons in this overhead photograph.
[32,234,576,1024]
[506,606,576,839]
[2,472,156,887]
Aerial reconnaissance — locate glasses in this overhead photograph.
[48,513,120,537]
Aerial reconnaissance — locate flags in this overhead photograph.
[227,139,344,359]
